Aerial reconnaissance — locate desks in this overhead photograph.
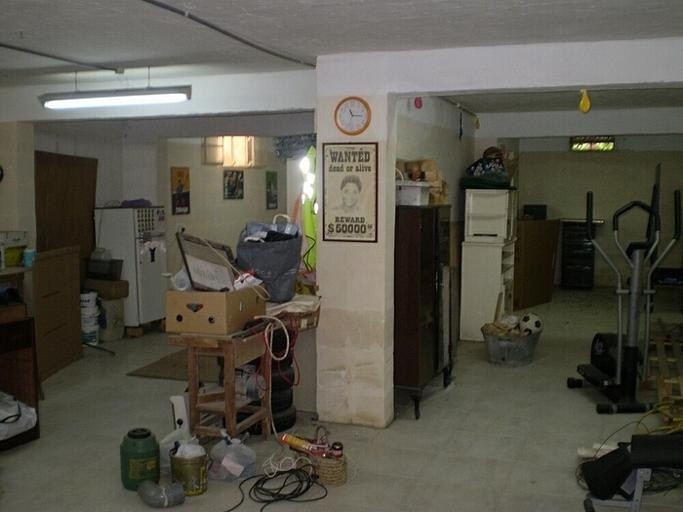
[168,312,276,440]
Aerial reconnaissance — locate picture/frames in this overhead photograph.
[323,143,378,243]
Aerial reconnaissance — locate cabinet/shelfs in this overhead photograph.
[464,188,521,243]
[460,242,515,341]
[395,203,453,420]
[0,315,40,453]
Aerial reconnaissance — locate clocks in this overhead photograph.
[334,96,371,136]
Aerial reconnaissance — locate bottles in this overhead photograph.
[330,442,343,459]
[281,434,314,454]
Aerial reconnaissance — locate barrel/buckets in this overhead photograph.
[80,292,102,347]
[120,427,161,490]
[481,331,541,365]
[170,448,208,497]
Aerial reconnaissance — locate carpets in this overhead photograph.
[126,349,220,383]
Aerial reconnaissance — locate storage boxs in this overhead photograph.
[83,257,124,281]
[165,282,266,336]
[396,180,433,206]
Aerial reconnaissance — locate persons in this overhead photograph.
[331,172,366,215]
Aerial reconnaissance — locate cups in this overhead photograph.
[22,248,34,267]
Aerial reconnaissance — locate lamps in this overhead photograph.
[37,67,191,110]
[570,136,613,152]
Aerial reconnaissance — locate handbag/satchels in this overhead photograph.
[235,213,304,306]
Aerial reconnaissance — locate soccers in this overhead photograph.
[518,312,544,335]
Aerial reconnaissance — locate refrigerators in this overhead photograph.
[93,205,169,327]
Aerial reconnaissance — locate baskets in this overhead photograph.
[296,426,348,487]
